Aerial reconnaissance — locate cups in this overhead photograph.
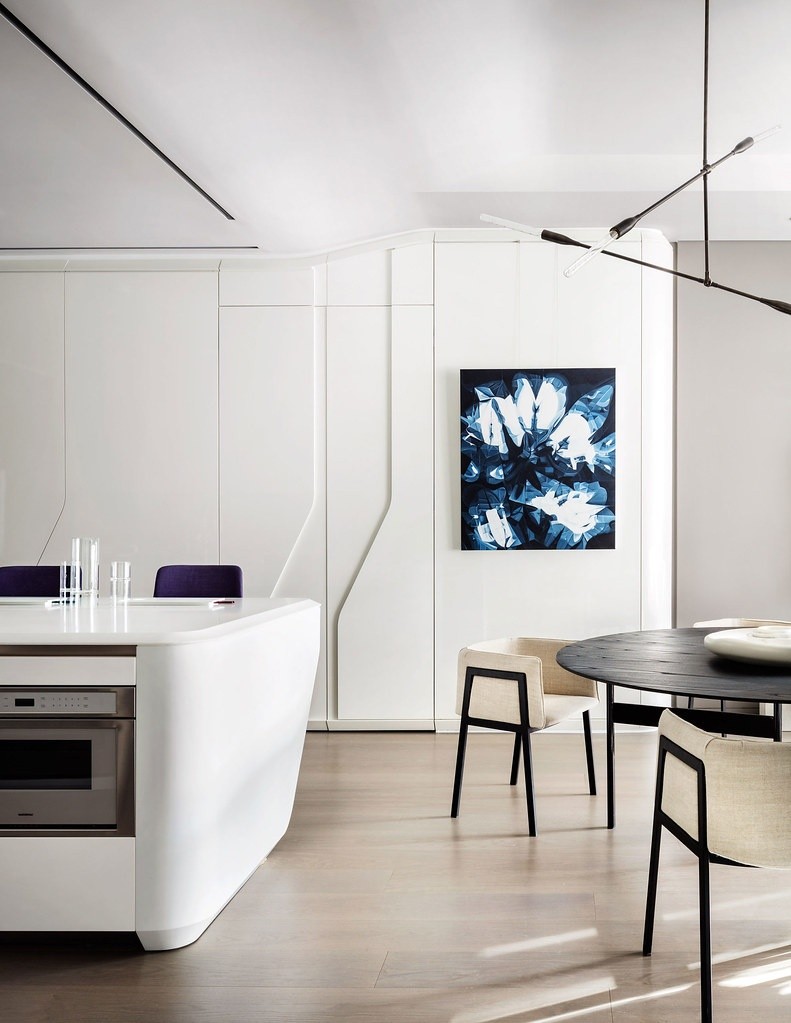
[60,561,81,604]
[110,561,131,598]
[71,537,99,600]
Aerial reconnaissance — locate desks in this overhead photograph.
[556,626,791,829]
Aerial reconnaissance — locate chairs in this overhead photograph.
[1,566,82,604]
[451,618,791,1023]
[152,565,244,598]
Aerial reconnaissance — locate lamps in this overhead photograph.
[478,0,790,315]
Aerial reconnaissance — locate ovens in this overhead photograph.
[0,686,136,838]
